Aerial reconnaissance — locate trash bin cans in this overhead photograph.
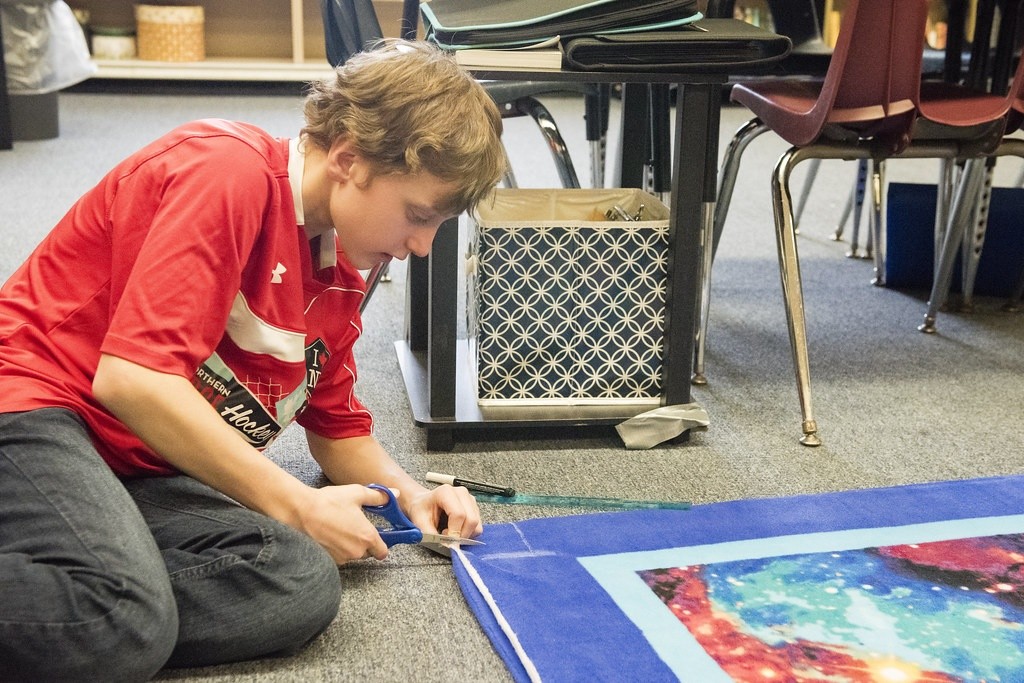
[0,0,92,143]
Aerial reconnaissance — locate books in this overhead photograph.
[453,34,563,68]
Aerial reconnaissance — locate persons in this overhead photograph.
[0,34,510,683]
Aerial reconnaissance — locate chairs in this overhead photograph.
[322,0,591,190]
[694,0,1024,451]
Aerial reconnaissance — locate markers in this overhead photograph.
[424,470,516,497]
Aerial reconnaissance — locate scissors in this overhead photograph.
[360,484,487,558]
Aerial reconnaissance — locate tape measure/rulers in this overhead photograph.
[467,490,693,512]
